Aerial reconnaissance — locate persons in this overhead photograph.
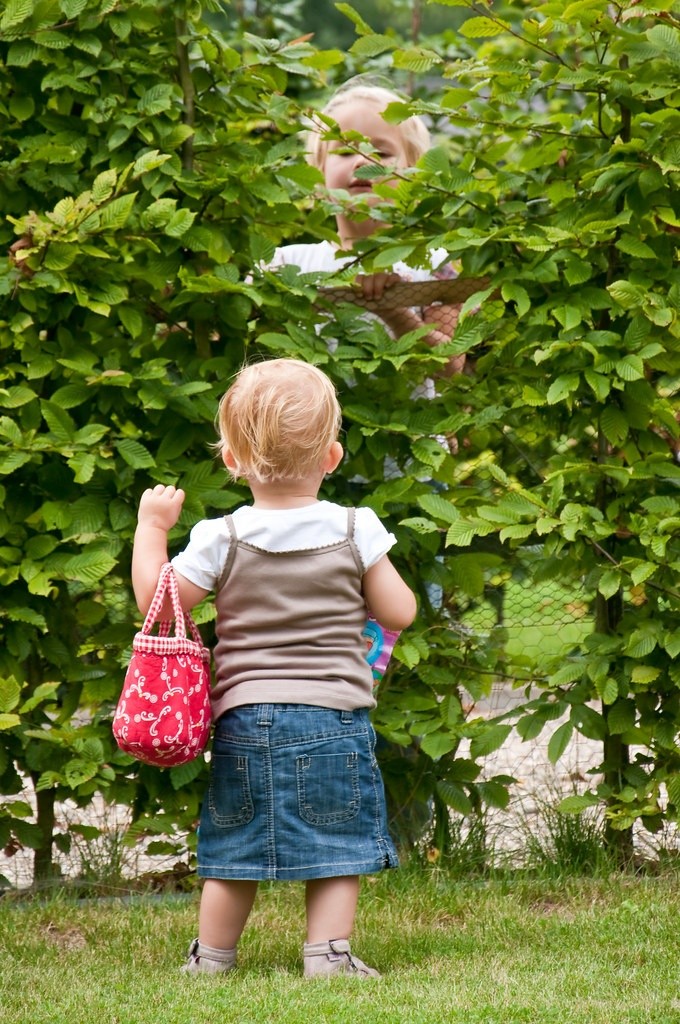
[131,357,418,977]
[244,72,474,722]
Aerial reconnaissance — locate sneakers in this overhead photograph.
[179,938,238,974]
[304,938,379,982]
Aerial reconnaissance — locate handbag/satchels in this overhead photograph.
[111,563,213,769]
